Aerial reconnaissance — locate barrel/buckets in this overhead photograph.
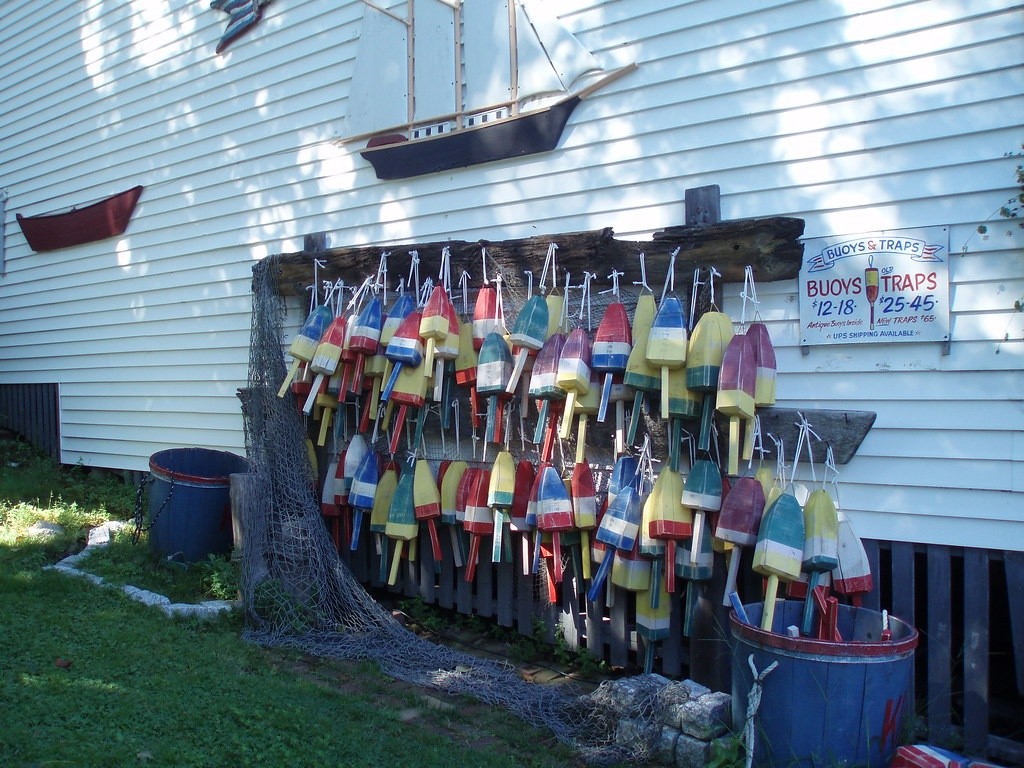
[148,447,252,563]
[728,599,919,768]
